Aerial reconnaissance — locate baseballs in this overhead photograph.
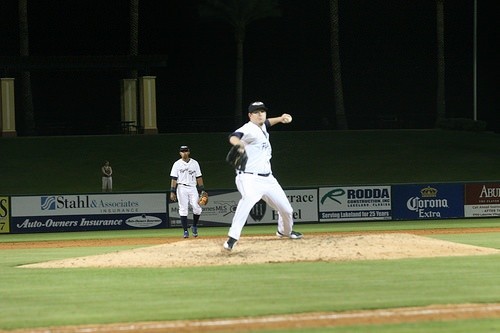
[285,115,292,123]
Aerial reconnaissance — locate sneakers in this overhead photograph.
[192,226,198,236]
[184,230,189,237]
[223,237,238,251]
[276,231,302,238]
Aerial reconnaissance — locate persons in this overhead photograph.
[170,146,208,239]
[222,102,303,251]
[101,161,112,192]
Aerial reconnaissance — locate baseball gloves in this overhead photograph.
[228,144,249,172]
[198,191,209,206]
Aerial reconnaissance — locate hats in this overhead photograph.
[179,146,190,152]
[248,102,268,113]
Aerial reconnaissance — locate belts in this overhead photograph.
[177,183,190,186]
[239,172,270,177]
